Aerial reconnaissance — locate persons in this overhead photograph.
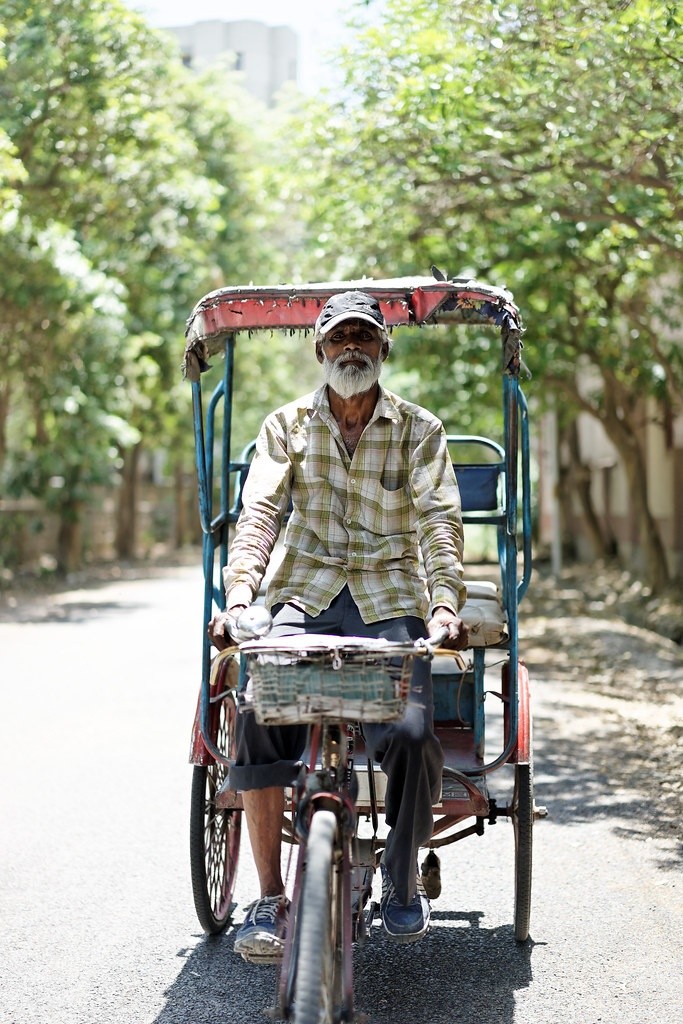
[207,289,470,954]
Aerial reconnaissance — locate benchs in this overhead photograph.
[221,562,506,648]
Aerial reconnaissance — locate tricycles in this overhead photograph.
[181,278,536,1024]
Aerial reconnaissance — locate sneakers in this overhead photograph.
[232,894,292,955]
[380,861,431,944]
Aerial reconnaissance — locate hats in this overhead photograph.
[314,290,385,338]
